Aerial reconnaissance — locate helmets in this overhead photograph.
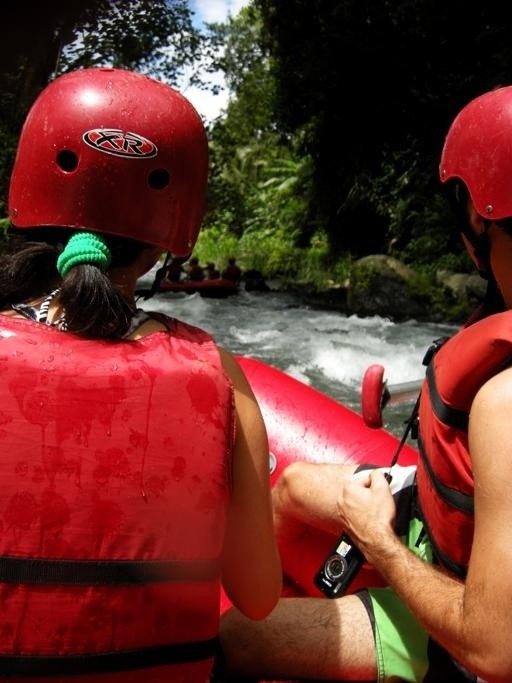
[440,85,512,222]
[8,68,207,255]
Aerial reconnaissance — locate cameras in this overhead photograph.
[314,532,364,600]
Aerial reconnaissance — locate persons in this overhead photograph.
[209,87,511,683]
[1,67,282,683]
